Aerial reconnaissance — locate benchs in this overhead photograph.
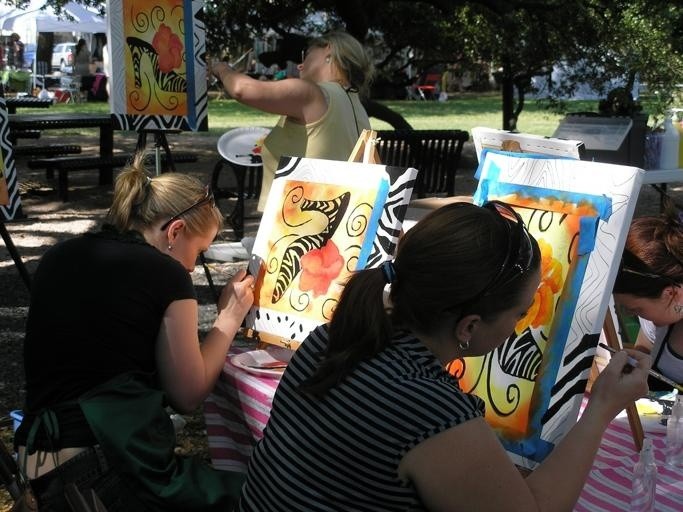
[6,130,197,203]
[209,130,469,242]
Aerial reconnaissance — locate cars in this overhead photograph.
[49,41,79,75]
[412,73,444,101]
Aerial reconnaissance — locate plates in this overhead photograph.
[215,125,273,169]
[227,346,297,381]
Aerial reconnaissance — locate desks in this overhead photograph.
[203,346,683,511]
[4,97,114,187]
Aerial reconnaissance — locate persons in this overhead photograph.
[612,198,683,396]
[236,201,658,512]
[210,30,383,213]
[439,70,448,102]
[11,151,256,512]
[404,72,422,102]
[72,38,96,102]
[11,32,25,70]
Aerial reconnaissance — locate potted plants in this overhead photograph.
[572,19,681,169]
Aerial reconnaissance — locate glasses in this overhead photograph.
[616,257,682,291]
[161,182,216,233]
[438,200,534,313]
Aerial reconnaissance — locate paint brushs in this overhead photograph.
[598,342,683,393]
[207,46,254,91]
[204,261,234,278]
